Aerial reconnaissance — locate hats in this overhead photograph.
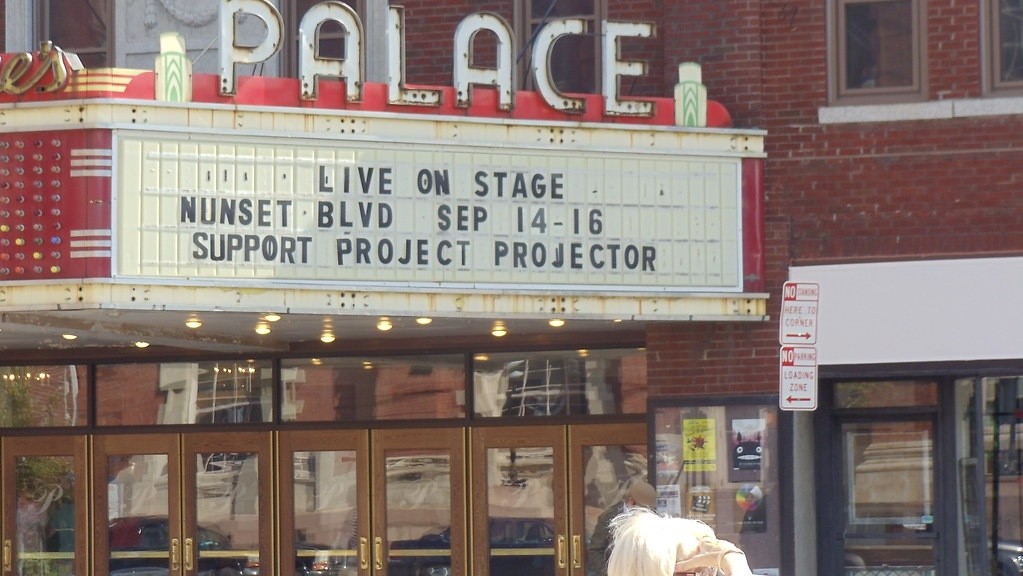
[629,483,656,507]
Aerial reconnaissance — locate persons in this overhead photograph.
[606,506,752,576]
[588,482,657,576]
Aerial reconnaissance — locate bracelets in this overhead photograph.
[717,548,744,575]
